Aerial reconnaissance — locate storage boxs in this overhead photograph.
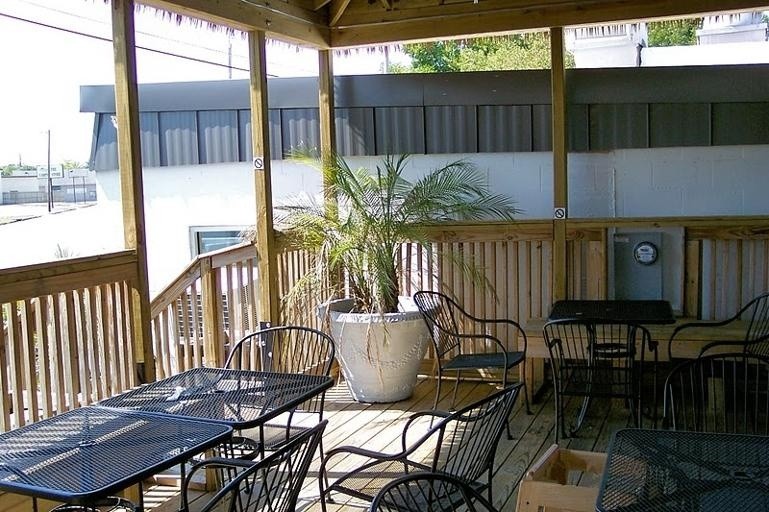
[515,448,647,512]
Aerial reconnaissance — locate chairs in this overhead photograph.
[177,326,335,495]
[149,419,328,512]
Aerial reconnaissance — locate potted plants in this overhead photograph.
[277,141,527,405]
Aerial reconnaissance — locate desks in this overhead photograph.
[595,428,769,511]
[93,368,334,512]
[0,407,233,512]
